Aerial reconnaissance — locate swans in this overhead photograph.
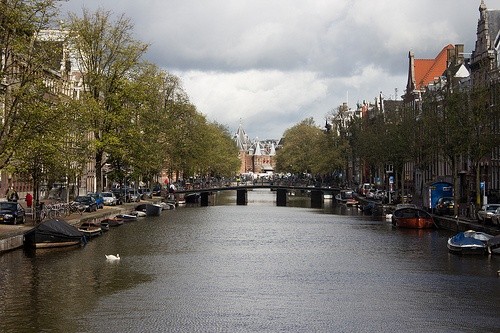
[105,254,120,260]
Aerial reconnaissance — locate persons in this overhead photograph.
[236,173,241,186]
[25,192,33,208]
[5,185,19,202]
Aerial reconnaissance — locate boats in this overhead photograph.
[391,203,438,231]
[447,229,497,257]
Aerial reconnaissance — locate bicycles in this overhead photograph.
[34,201,83,222]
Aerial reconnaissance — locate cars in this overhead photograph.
[0,201,26,225]
[355,182,414,205]
[86,191,105,209]
[100,191,117,206]
[69,195,98,213]
[490,207,500,230]
[476,204,500,225]
[435,196,455,217]
[111,176,218,205]
